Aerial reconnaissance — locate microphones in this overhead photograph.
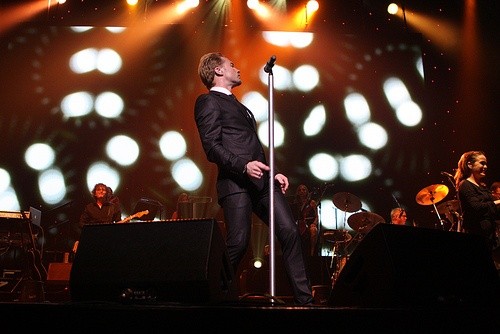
[441,172,453,178]
[265,56,276,72]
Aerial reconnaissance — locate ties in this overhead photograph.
[229,94,238,101]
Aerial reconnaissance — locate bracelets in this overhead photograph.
[243,164,247,173]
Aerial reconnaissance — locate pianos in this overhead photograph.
[0,207,42,226]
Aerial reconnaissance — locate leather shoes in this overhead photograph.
[303,301,324,307]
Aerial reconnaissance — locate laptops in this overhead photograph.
[29,207,41,226]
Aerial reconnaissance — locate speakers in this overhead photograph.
[71,217,238,304]
[332,222,493,309]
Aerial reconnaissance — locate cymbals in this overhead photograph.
[333,191,362,212]
[416,183,450,206]
[347,211,385,234]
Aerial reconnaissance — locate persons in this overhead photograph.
[79,184,129,229]
[391,208,407,225]
[454,151,500,248]
[172,194,189,219]
[192,51,325,307]
[291,184,319,256]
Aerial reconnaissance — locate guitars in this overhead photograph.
[115,209,149,223]
